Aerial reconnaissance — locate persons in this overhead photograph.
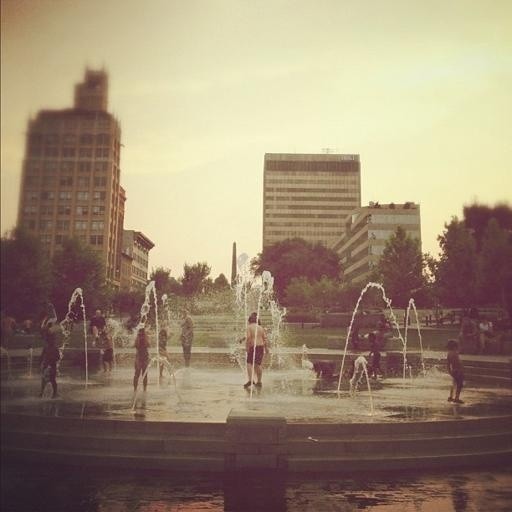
[1,314,36,347]
[88,310,106,346]
[158,322,176,379]
[237,312,268,388]
[314,311,401,389]
[445,341,465,404]
[239,312,262,343]
[98,333,114,373]
[37,337,60,400]
[133,328,149,393]
[451,304,509,354]
[179,308,195,364]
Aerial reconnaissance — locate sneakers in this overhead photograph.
[245,380,263,387]
[447,397,464,403]
